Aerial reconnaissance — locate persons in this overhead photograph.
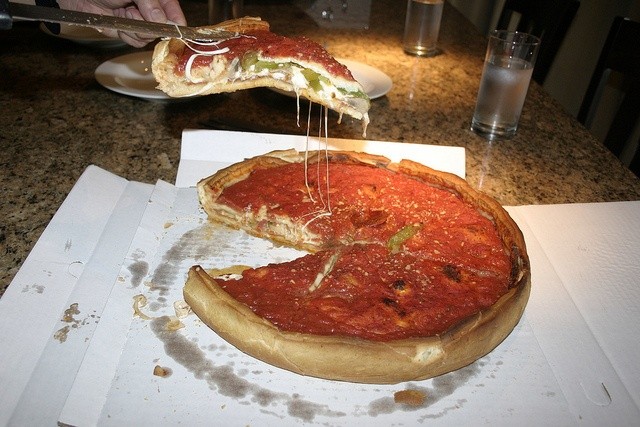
[0,0,187,48]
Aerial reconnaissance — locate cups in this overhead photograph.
[471,30,541,142]
[402,0,444,57]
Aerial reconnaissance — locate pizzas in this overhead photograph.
[150,15,371,139]
[181,148,531,385]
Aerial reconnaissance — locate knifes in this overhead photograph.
[0,1,231,40]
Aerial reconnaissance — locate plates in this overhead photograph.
[333,58,393,101]
[40,21,128,48]
[93,50,178,100]
